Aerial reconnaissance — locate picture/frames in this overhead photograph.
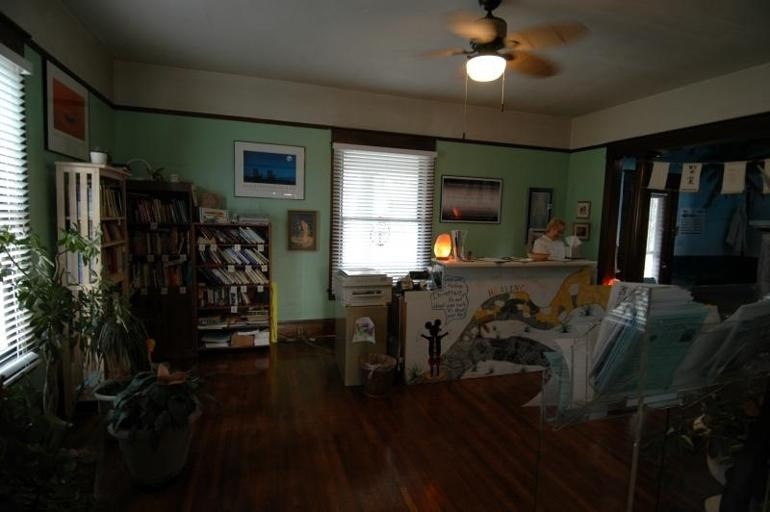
[439,175,503,226]
[287,211,318,250]
[526,187,554,243]
[573,201,591,239]
[233,142,305,199]
[41,58,90,162]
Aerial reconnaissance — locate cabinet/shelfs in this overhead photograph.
[125,176,196,365]
[198,215,270,351]
[55,165,132,378]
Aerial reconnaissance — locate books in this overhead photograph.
[100,184,127,275]
[132,198,270,348]
[588,282,721,393]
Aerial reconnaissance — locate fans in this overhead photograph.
[420,0,589,79]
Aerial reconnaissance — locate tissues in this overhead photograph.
[564,234,582,257]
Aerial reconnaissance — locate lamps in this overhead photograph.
[463,43,510,83]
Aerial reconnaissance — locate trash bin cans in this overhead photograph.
[359,352,398,399]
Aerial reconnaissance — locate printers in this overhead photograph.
[332,269,394,308]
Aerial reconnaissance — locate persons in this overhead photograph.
[533,217,566,261]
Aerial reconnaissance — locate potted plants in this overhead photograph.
[89,144,108,164]
[1,225,206,489]
